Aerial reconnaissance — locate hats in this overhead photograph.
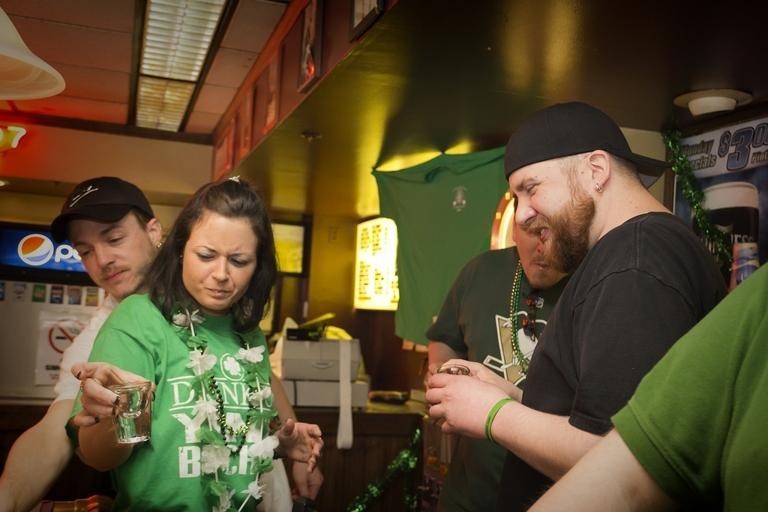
[51,176,154,245]
[504,101,675,190]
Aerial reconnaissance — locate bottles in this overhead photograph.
[687,180,760,298]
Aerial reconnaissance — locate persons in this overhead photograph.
[422,100,729,509]
[68,175,326,512]
[423,191,578,511]
[1,173,326,512]
[523,257,766,511]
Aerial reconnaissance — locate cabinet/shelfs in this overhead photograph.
[271,388,423,512]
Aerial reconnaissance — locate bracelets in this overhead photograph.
[483,397,518,449]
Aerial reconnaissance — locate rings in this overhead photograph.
[79,375,99,394]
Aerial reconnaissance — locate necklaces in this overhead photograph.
[509,257,531,373]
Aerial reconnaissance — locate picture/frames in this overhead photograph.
[199,1,388,179]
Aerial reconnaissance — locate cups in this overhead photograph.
[107,380,152,444]
